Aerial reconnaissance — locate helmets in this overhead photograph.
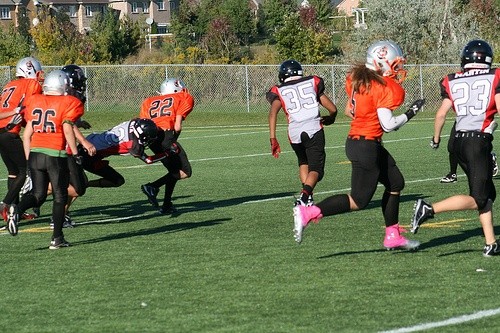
[15,57,42,79]
[62,65,84,92]
[160,77,187,95]
[278,60,303,83]
[42,70,70,95]
[132,118,158,144]
[461,40,494,69]
[365,40,404,77]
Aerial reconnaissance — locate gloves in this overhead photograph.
[270,138,281,159]
[25,160,31,177]
[72,153,84,165]
[430,136,441,149]
[405,98,426,120]
[320,115,335,125]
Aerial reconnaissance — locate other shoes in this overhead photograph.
[33,208,40,216]
[20,176,33,198]
[21,211,35,219]
[50,211,76,229]
[0,201,11,222]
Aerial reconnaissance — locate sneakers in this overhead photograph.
[159,202,179,215]
[410,199,434,234]
[6,204,24,237]
[49,234,71,250]
[440,172,458,184]
[491,152,499,177]
[483,239,500,257]
[293,194,313,208]
[140,182,160,209]
[292,205,323,244]
[384,224,420,250]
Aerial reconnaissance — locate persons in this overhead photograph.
[0,56,194,249]
[410,39,500,257]
[293,40,424,252]
[266,60,338,207]
